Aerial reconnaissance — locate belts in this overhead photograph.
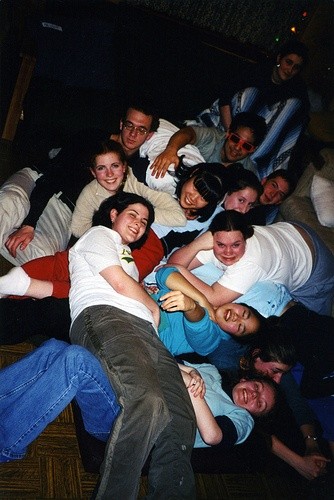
[29,164,75,213]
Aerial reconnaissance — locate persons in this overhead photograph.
[68,192,197,500]
[0,42,334,481]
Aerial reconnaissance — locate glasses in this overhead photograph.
[125,121,150,135]
[228,133,254,152]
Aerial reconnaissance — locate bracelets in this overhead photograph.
[305,434,318,440]
